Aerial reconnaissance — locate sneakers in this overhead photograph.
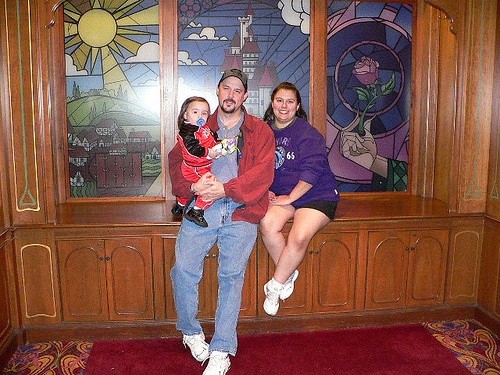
[201,353,231,375]
[280,269,299,299]
[182,331,211,362]
[171,204,183,216]
[263,280,280,317]
[185,208,208,227]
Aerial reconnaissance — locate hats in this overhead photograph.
[218,69,247,91]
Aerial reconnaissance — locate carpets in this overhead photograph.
[82,322,473,375]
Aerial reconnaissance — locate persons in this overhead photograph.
[171,96,220,228]
[168,69,275,375]
[260,83,339,317]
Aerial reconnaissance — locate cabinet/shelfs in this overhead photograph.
[55,196,487,322]
